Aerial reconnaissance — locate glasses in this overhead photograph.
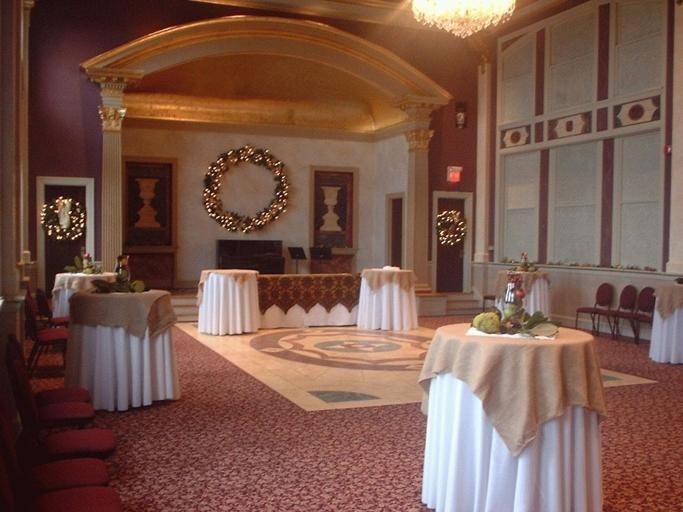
[410,0,516,38]
[455,101,467,129]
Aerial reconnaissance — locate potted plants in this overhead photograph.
[122,153,177,255]
[309,163,358,255]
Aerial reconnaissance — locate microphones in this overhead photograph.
[482,295,495,312]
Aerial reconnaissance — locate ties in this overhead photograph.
[217,238,283,274]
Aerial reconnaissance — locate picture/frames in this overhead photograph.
[494,270,552,322]
[418,322,609,512]
[49,271,118,317]
[257,273,360,328]
[195,268,261,336]
[64,289,182,411]
[648,280,683,365]
[357,268,419,332]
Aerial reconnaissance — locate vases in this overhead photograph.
[469,274,563,337]
[39,195,87,241]
[90,253,145,293]
[202,142,290,234]
[511,250,539,272]
[63,251,103,274]
[436,210,468,247]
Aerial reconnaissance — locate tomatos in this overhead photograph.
[216,240,285,274]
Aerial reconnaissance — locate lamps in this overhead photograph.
[575,282,655,345]
[0,287,127,512]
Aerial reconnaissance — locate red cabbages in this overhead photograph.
[130,280,144,292]
[472,312,500,334]
